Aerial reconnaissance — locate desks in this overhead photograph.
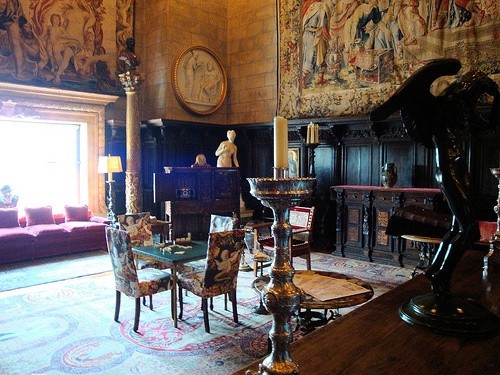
[231,248,500,375]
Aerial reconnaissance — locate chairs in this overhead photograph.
[183,213,239,311]
[118,211,173,306]
[176,229,245,332]
[104,226,175,332]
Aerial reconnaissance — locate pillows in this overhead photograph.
[64,205,89,221]
[25,207,55,226]
[0,207,18,228]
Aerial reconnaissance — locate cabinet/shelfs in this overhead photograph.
[164,166,241,242]
[332,186,451,270]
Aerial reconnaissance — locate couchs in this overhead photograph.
[0,204,111,264]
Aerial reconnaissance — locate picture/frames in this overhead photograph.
[171,44,228,117]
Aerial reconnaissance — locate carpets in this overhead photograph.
[0,245,425,375]
[0,249,114,293]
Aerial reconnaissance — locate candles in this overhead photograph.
[107,156,112,181]
[307,122,319,144]
[273,116,289,168]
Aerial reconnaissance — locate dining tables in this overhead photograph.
[131,240,213,319]
